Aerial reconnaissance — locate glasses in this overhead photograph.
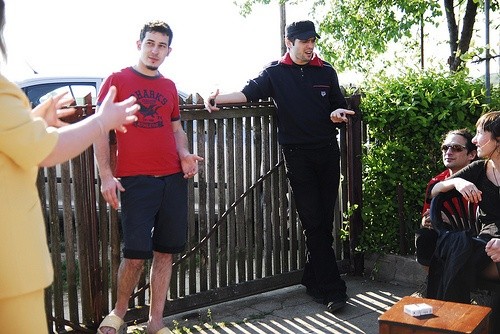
[441,144,466,152]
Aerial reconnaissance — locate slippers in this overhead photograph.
[97,308,125,334]
[155,327,173,334]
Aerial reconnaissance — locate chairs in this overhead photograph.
[430,187,499,272]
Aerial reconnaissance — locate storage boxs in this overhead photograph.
[404,303,433,317]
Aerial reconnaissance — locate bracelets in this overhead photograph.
[89,116,104,136]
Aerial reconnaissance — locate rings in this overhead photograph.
[469,189,474,193]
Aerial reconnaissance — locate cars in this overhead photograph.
[17,74,289,246]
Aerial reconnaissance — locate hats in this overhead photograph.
[285,20,320,41]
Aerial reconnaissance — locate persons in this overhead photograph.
[420,111,500,334]
[92,20,203,334]
[204,21,355,312]
[0,0,140,334]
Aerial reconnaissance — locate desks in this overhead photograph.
[377,296,491,334]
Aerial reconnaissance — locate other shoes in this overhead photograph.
[327,294,349,313]
[306,286,328,304]
